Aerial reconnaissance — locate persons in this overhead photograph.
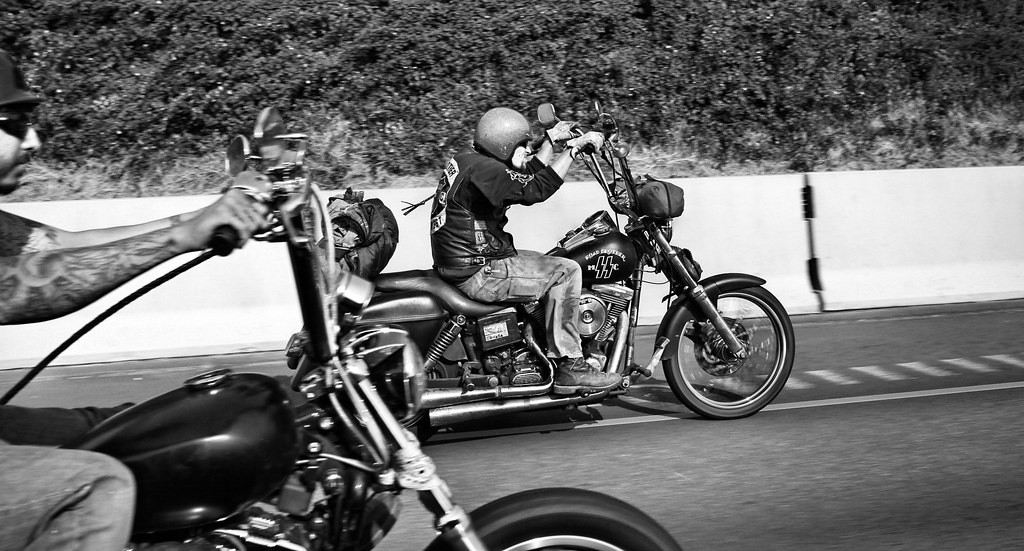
[430,108,624,394]
[0,49,268,551]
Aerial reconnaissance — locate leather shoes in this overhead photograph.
[552,357,622,394]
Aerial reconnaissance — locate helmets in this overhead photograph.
[474,107,533,160]
[0,47,48,105]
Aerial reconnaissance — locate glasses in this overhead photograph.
[0,112,29,141]
[518,138,528,148]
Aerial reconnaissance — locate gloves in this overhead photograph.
[545,119,582,146]
[566,131,605,160]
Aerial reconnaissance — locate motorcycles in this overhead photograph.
[0,106,686,551]
[284,99,796,454]
